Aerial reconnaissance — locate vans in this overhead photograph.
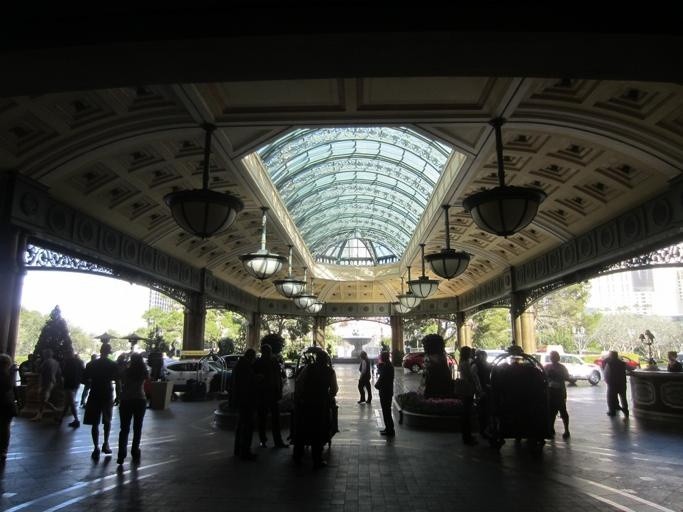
[179,350,209,360]
[217,354,261,376]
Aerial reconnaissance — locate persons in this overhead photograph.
[666,351,683,372]
[602,350,629,422]
[226,344,339,462]
[453,346,577,446]
[0,341,164,494]
[357,351,372,403]
[374,351,395,436]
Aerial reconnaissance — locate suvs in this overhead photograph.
[528,353,602,386]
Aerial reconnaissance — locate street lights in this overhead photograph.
[96,333,117,345]
[121,333,147,353]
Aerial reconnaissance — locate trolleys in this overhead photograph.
[286,348,340,457]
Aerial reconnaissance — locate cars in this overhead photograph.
[284,362,298,380]
[677,354,683,365]
[595,354,638,375]
[402,351,456,373]
[482,348,511,368]
[147,359,230,392]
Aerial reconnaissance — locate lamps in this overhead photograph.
[163,122,245,240]
[423,205,471,280]
[392,276,412,313]
[462,118,547,239]
[272,244,307,298]
[406,243,439,299]
[304,277,323,313]
[294,267,318,310]
[239,207,287,281]
[396,266,422,308]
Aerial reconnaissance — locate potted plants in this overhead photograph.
[144,326,174,410]
